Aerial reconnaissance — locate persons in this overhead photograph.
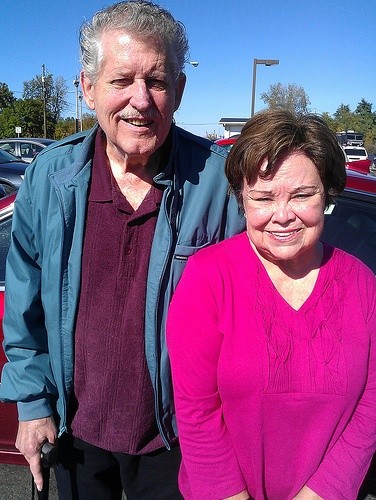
[0,0,246,500]
[166,107,376,500]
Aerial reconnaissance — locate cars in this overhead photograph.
[0,137,68,469]
[217,136,376,275]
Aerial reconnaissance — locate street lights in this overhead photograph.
[251,58,280,117]
